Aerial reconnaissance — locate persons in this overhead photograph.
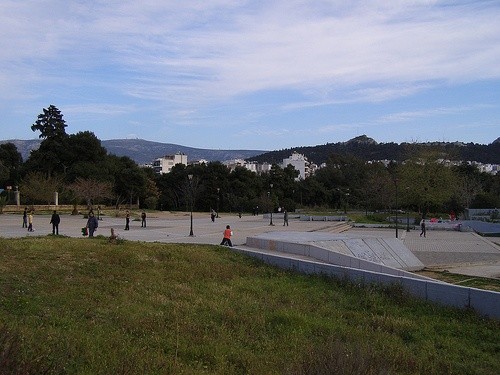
[211,209,217,222]
[124,210,130,230]
[22,207,28,228]
[27,206,35,213]
[283,210,288,226]
[252,206,258,216]
[140,212,146,227]
[219,224,233,247]
[420,219,426,238]
[86,211,98,238]
[274,206,284,213]
[50,211,60,235]
[27,213,33,232]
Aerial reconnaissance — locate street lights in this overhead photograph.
[216,187,221,218]
[269,182,273,225]
[392,178,400,238]
[187,173,194,236]
